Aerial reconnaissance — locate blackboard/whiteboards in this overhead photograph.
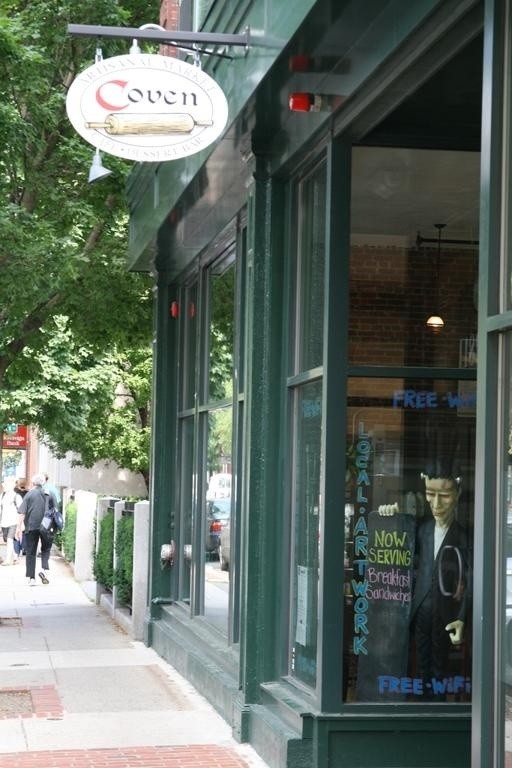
[355,510,416,702]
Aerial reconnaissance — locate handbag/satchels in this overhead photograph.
[39,508,63,546]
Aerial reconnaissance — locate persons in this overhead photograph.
[0,475,24,565]
[12,473,62,558]
[376,457,472,700]
[14,469,60,587]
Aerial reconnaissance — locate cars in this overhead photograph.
[192,470,235,573]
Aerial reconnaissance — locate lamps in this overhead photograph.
[425,224,448,333]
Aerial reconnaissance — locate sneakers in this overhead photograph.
[28,579,35,585]
[39,573,49,585]
